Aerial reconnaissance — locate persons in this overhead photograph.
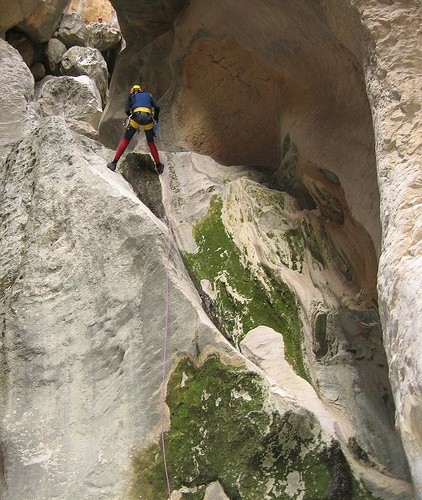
[107,84,164,174]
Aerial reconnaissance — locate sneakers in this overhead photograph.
[107,160,116,170]
[156,163,165,173]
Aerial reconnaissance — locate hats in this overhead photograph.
[130,84,142,92]
